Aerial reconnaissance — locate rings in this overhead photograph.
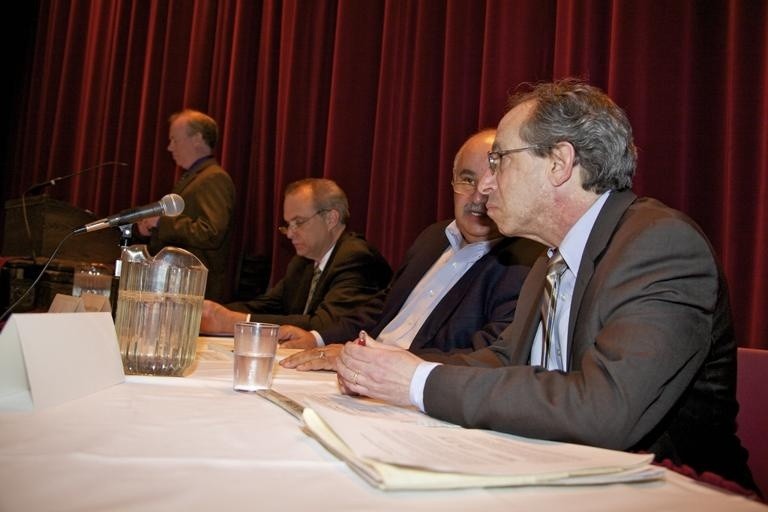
[351,373,359,385]
[319,351,326,360]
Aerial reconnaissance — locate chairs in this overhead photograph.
[732,348,767,498]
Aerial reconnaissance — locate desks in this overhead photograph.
[1,337,768,512]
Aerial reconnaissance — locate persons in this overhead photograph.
[335,76,760,499]
[276,127,545,372]
[139,108,237,302]
[200,175,392,342]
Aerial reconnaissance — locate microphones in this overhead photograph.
[73,193,185,237]
[24,160,129,194]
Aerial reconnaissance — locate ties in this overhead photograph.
[303,267,321,316]
[541,250,568,371]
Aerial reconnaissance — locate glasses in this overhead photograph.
[451,175,477,196]
[278,209,332,234]
[488,146,553,171]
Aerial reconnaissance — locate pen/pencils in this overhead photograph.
[358,333,366,346]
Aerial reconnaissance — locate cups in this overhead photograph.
[233,322,279,392]
[72,261,114,299]
[114,244,209,378]
